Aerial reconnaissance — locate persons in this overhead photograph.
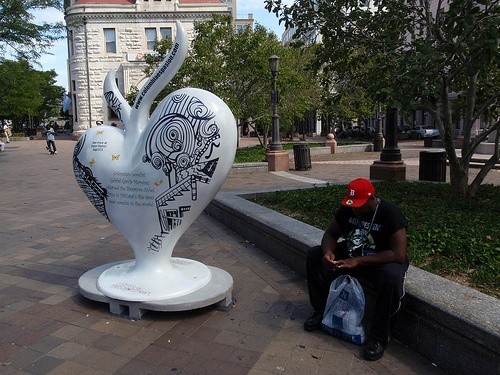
[97,122,101,126]
[12,121,69,136]
[3,122,11,143]
[304,177,409,361]
[44,125,58,154]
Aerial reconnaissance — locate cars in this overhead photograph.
[405,125,440,140]
[397,125,412,133]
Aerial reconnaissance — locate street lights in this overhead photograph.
[267,54,286,153]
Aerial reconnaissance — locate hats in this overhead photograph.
[340,178,376,209]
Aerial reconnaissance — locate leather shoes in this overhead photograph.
[303,310,323,330]
[362,337,390,361]
